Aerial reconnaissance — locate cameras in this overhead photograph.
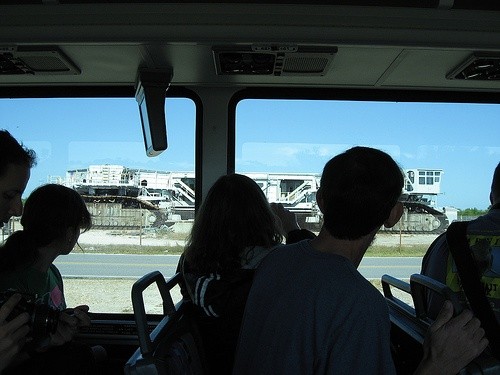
[2,288,58,347]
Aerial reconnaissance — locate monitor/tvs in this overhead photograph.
[135,79,167,158]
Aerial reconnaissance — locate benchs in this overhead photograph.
[125,271,219,375]
[381,273,468,375]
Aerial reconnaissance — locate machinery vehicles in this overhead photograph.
[46,165,450,236]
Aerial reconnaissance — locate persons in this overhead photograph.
[0,128,92,372]
[175,174,317,375]
[421,161,500,375]
[0,184,107,375]
[233,147,489,375]
